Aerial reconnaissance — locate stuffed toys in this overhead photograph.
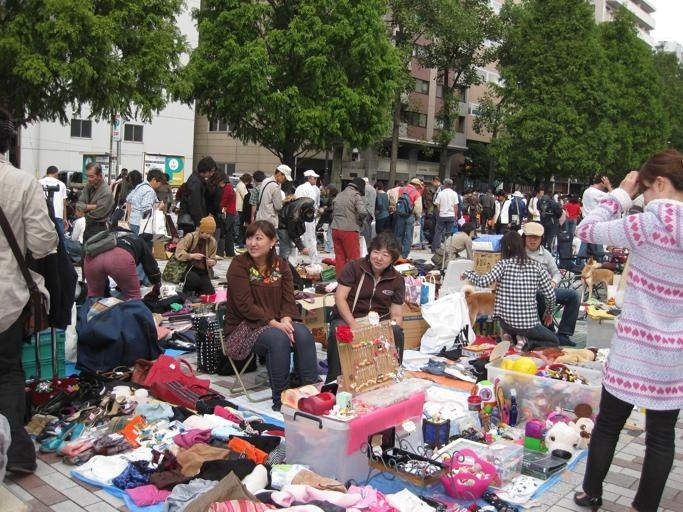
[544,420,581,460]
[568,417,594,449]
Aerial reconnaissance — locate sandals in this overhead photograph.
[26,378,138,457]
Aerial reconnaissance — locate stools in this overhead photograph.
[474,250,503,288]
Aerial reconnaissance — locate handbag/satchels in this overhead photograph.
[85,229,118,258]
[162,254,190,285]
[74,296,166,379]
[512,214,520,225]
[432,248,448,267]
[422,276,436,305]
[26,289,48,337]
[132,355,239,414]
[191,316,224,373]
[138,207,179,244]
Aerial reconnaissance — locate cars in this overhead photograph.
[59,172,81,187]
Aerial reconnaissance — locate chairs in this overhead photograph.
[587,243,629,274]
[552,277,572,328]
[217,301,272,403]
[554,239,582,289]
[322,292,335,339]
[583,281,604,302]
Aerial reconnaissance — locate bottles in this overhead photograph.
[468,395,482,411]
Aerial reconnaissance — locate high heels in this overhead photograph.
[574,492,602,512]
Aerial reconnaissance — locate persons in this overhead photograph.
[223,220,317,411]
[0,106,59,474]
[460,231,559,352]
[573,149,682,512]
[323,229,404,384]
[38,157,645,303]
[520,222,580,346]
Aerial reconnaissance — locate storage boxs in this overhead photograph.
[402,311,431,350]
[294,290,335,331]
[280,377,426,485]
[480,437,524,487]
[585,314,615,348]
[485,355,602,424]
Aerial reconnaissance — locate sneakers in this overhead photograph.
[9,467,34,474]
[560,336,575,345]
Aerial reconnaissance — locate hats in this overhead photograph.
[511,191,523,197]
[200,216,216,234]
[276,165,292,182]
[411,178,423,189]
[523,222,544,237]
[349,178,366,195]
[304,170,319,177]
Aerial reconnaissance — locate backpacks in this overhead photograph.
[396,188,415,217]
